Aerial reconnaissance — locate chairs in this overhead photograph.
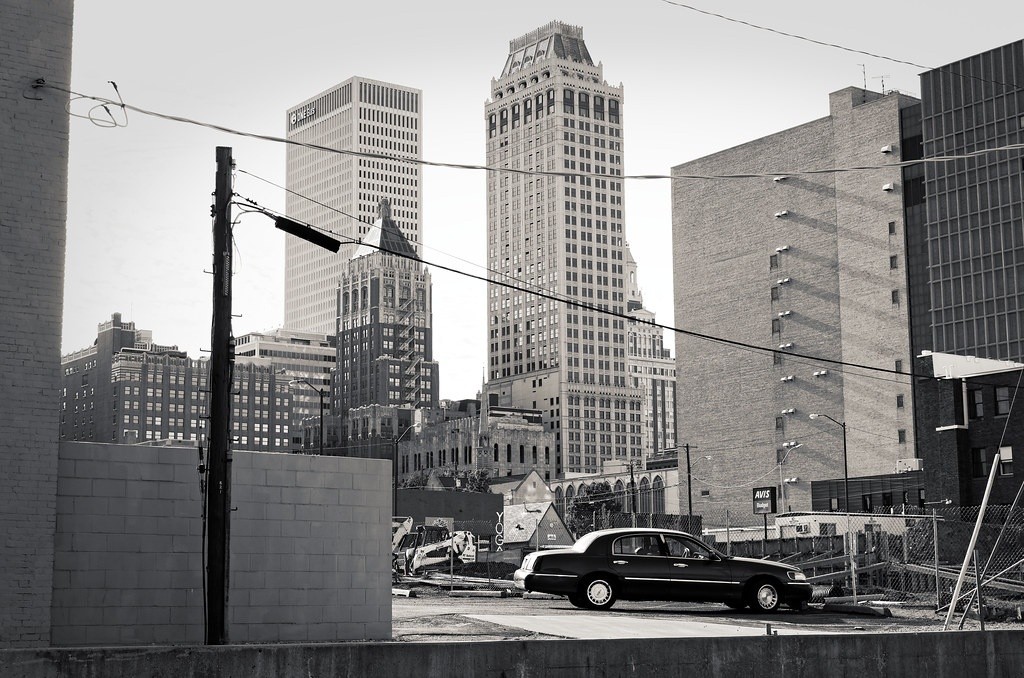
[648,544,659,555]
[635,546,647,554]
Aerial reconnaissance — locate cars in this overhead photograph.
[513,527,812,619]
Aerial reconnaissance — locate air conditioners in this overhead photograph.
[795,524,808,534]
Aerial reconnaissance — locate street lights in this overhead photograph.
[808,414,850,514]
[289,379,327,456]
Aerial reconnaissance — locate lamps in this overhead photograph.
[781,375,794,381]
[883,183,893,193]
[782,210,789,215]
[814,370,827,376]
[774,177,780,181]
[782,408,795,414]
[783,245,791,251]
[774,212,782,217]
[779,342,794,350]
[777,278,790,285]
[881,145,893,154]
[779,311,792,318]
[784,478,798,483]
[776,247,782,253]
[781,175,788,179]
[783,441,797,447]
